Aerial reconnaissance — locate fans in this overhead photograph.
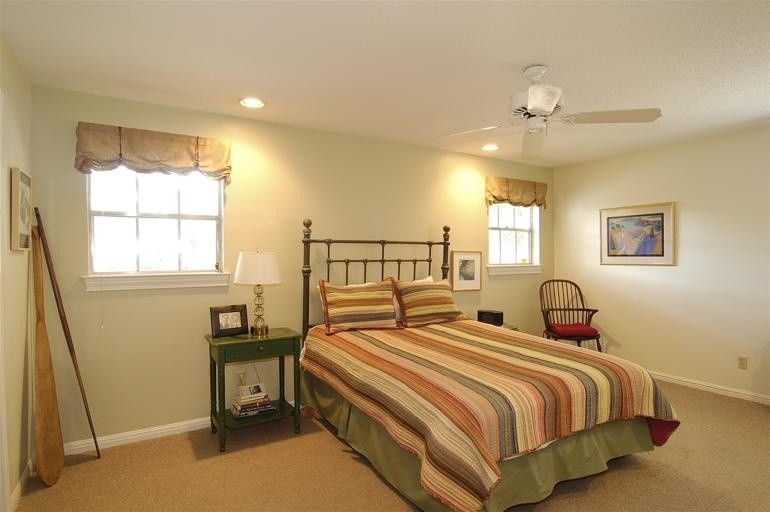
[441,64,664,150]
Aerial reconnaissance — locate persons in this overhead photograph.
[220,314,231,329]
[232,314,241,328]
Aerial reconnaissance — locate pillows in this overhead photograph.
[318,276,398,335]
[394,274,435,322]
[397,277,475,328]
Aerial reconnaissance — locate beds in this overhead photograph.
[301,216,681,512]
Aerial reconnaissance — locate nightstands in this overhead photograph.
[204,327,303,453]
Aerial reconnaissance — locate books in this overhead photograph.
[230,384,277,419]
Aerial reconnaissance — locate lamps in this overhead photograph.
[233,248,283,335]
[522,65,563,131]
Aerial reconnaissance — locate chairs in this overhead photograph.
[538,278,603,354]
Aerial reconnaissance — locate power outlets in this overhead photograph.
[736,356,749,370]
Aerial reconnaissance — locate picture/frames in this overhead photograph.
[450,249,484,291]
[11,167,34,250]
[210,304,250,338]
[598,202,678,267]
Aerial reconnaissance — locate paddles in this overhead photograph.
[30,225,64,486]
